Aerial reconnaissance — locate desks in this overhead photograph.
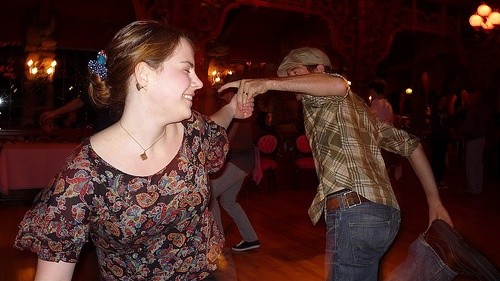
[0,127,93,196]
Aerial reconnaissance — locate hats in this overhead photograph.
[277,47,333,78]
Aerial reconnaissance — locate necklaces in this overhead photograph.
[120,121,165,160]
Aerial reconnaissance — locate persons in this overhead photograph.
[450,87,465,120]
[367,79,393,123]
[430,93,451,189]
[40,92,116,132]
[400,81,417,117]
[462,93,486,199]
[217,48,500,281]
[13,21,255,281]
[210,86,260,251]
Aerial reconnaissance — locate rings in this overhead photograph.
[243,92,248,95]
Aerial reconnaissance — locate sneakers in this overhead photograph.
[232,238,260,251]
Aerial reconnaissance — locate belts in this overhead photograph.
[326,191,367,211]
